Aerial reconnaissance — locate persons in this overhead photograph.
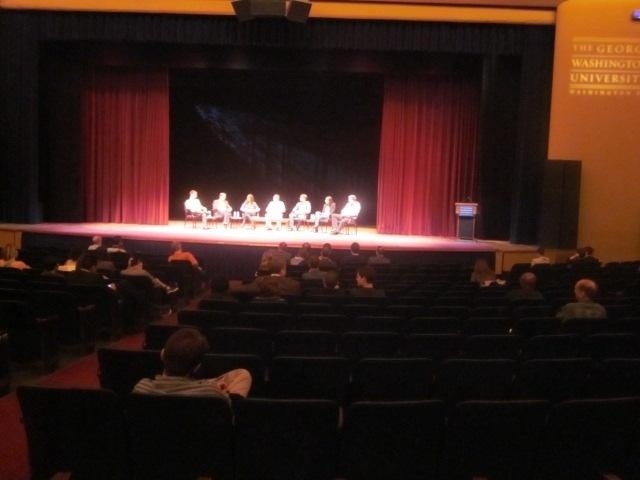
[184,190,209,230]
[54,236,178,318]
[289,194,311,231]
[133,329,254,401]
[309,197,335,231]
[331,195,361,234]
[213,193,232,229]
[207,240,390,300]
[167,241,202,271]
[470,246,606,319]
[265,194,286,230]
[2,241,31,270]
[240,194,261,229]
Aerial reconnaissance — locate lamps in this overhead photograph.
[230,1,313,23]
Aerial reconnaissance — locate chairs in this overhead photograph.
[1,253,640,480]
[183,203,359,236]
